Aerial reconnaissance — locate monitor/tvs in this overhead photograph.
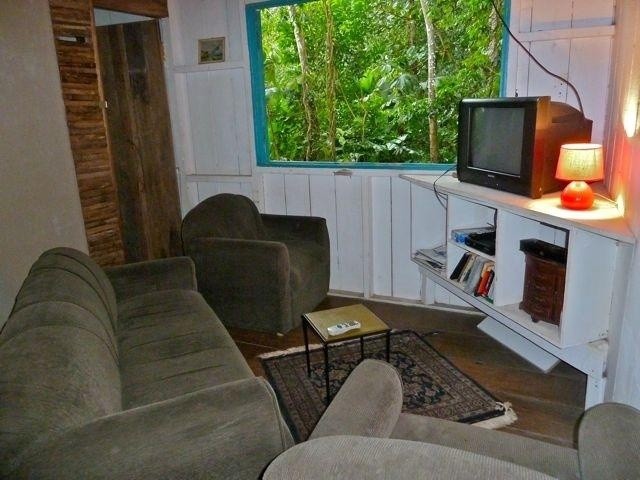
[456,96,593,200]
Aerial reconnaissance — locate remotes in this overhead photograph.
[326,319,362,337]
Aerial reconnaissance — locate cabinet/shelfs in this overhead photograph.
[403,174,635,410]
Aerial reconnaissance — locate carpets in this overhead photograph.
[257,327,517,442]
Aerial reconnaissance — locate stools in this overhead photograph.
[301,303,391,401]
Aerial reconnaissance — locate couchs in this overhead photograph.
[0,247,294,480]
[181,193,331,333]
[262,357,640,480]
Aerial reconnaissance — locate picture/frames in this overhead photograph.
[198,36,226,64]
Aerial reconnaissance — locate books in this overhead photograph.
[449,251,496,304]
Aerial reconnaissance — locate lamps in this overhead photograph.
[554,144,604,210]
